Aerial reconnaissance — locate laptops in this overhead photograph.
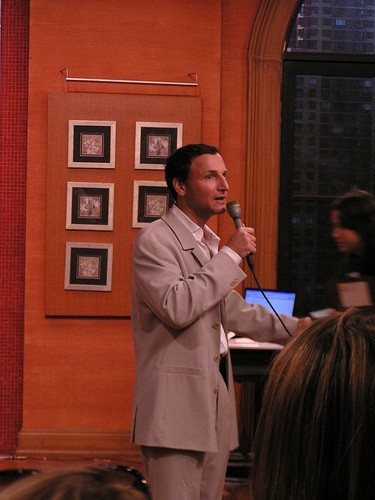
[244,288,298,318]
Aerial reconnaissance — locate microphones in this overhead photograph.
[227,200,255,270]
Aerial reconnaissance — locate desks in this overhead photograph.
[219,339,284,481]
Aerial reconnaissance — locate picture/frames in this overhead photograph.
[63,242,114,292]
[65,181,114,232]
[132,180,174,227]
[67,121,117,168]
[134,120,183,170]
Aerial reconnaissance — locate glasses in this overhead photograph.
[0,464,153,500]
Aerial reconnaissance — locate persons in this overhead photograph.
[0,463,152,500]
[250,305,375,500]
[331,187,375,310]
[131,144,312,499]
